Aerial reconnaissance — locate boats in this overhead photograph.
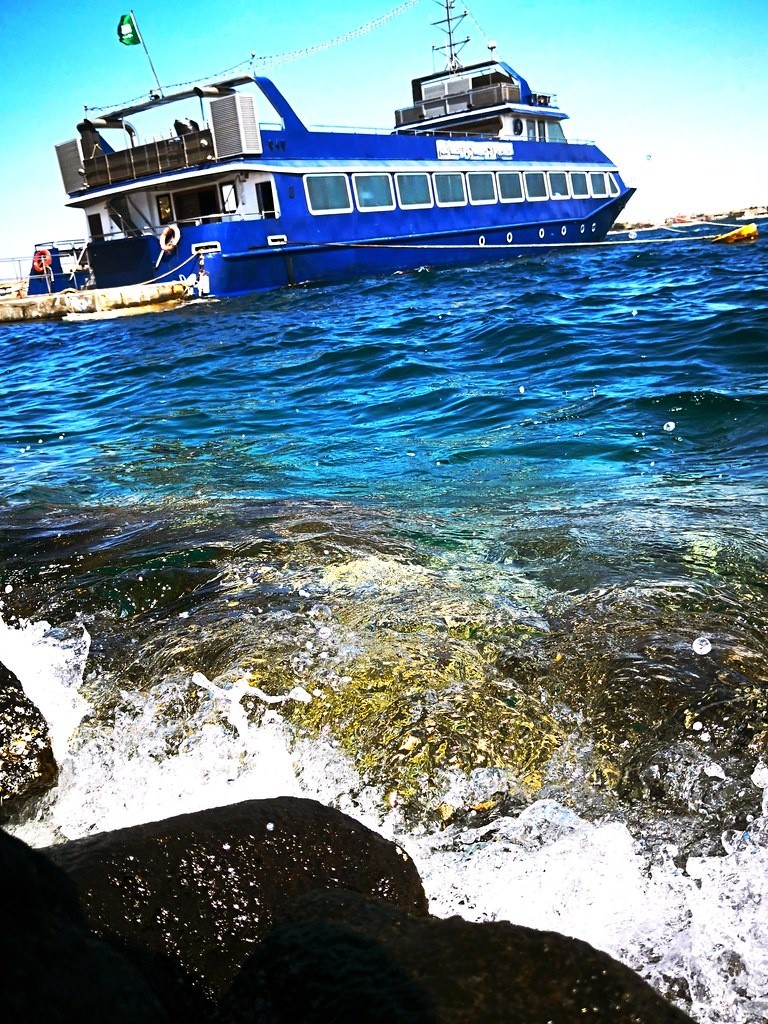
[0,0,637,322]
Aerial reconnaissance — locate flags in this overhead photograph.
[117,15,141,45]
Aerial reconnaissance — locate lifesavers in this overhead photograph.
[512,119,523,136]
[159,225,182,252]
[33,248,52,272]
